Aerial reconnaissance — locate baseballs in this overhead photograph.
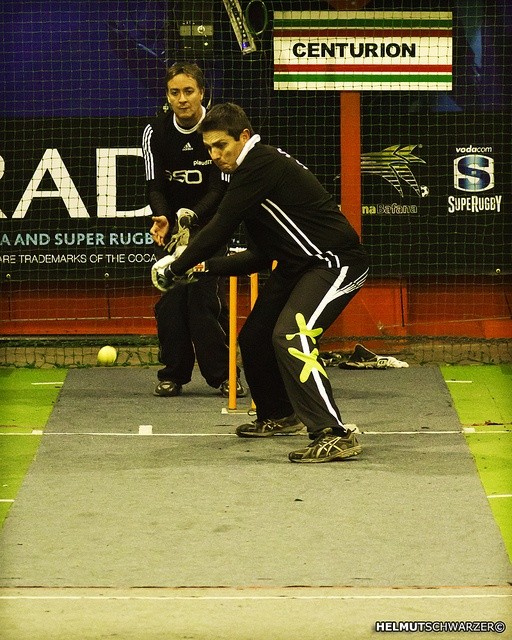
[97,345,117,366]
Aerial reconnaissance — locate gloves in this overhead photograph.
[147,247,201,293]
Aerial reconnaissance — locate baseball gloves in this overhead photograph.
[164,208,199,256]
[319,351,342,367]
[338,344,388,369]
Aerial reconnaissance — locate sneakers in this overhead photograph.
[288,428,362,463]
[154,381,182,397]
[220,378,247,398]
[236,410,305,438]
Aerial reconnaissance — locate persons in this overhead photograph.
[150,102,371,463]
[142,61,249,398]
